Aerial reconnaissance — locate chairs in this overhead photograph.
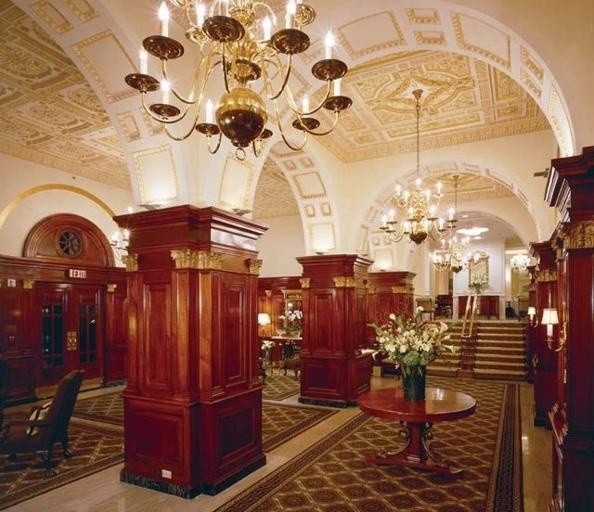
[263,340,301,378]
[0,369,84,477]
[416,295,453,320]
[516,281,529,321]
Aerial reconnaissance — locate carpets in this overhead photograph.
[71,390,340,453]
[262,375,301,401]
[0,412,125,510]
[213,377,525,512]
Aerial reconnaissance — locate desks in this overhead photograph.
[357,387,476,478]
[458,295,499,319]
[272,337,302,359]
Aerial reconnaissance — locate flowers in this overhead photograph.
[278,310,303,330]
[361,306,461,376]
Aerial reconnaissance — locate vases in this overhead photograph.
[287,330,300,338]
[400,362,426,402]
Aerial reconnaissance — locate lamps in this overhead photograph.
[528,307,539,328]
[542,302,567,352]
[433,176,481,273]
[379,88,457,245]
[124,0,354,161]
[258,313,271,339]
[510,253,531,272]
[109,207,132,256]
